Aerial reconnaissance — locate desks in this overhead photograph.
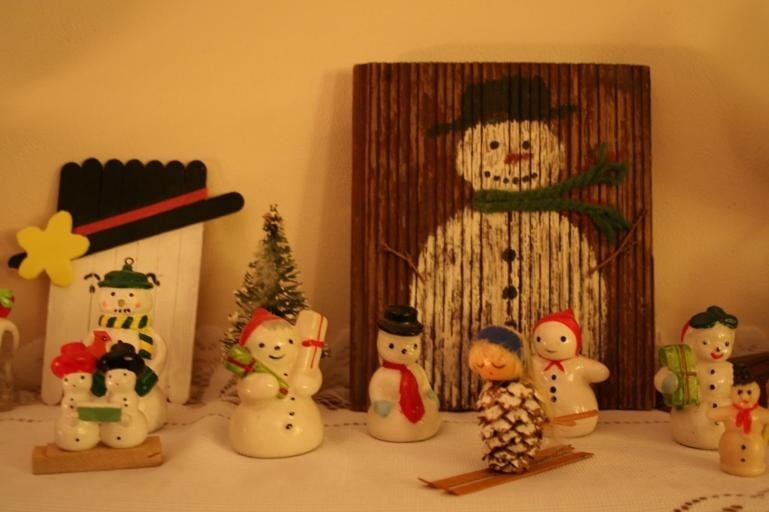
[0,404,769,512]
